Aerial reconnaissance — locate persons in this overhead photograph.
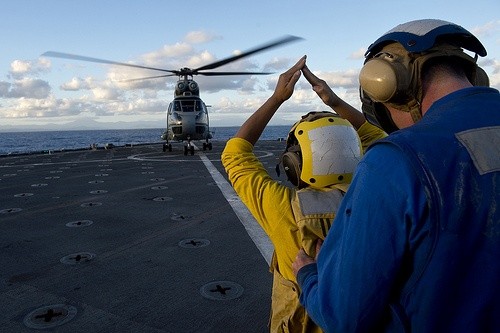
[221,55,389,333]
[291,19,500,333]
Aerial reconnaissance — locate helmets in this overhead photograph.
[359,19,489,134]
[276,112,364,188]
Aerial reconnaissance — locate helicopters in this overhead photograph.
[39,33,306,158]
